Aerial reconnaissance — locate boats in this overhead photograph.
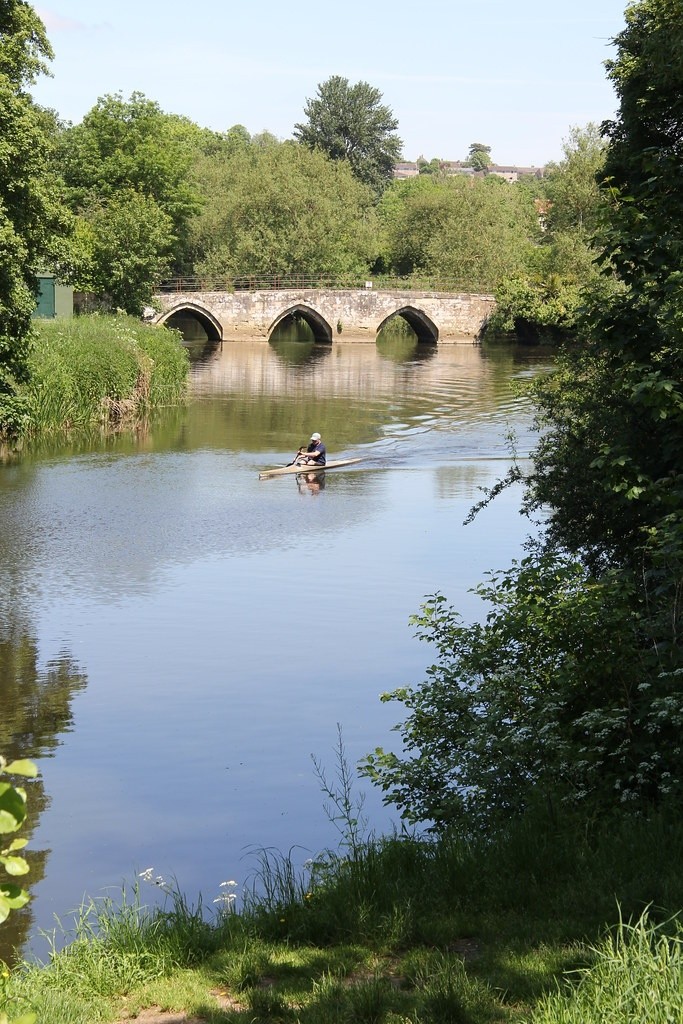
[258,457,362,479]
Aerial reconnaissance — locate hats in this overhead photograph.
[310,433,321,441]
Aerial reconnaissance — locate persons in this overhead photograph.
[297,432,326,466]
[296,470,325,496]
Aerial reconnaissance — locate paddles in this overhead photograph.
[285,446,306,467]
